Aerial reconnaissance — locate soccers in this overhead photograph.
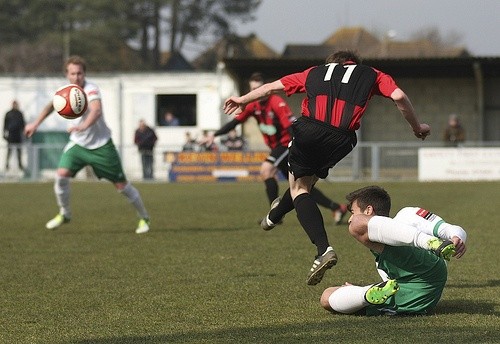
[51,83,89,120]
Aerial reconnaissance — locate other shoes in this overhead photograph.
[331,204,348,226]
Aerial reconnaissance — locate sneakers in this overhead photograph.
[45,212,71,230]
[426,237,455,262]
[260,196,284,232]
[135,216,151,234]
[363,278,400,305]
[306,246,337,286]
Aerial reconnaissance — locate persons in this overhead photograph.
[183,132,199,152]
[160,111,181,125]
[222,50,430,286]
[222,126,247,151]
[135,119,158,183]
[319,185,467,317]
[4,101,31,173]
[23,53,151,234]
[443,114,465,148]
[201,71,350,227]
[198,129,217,152]
